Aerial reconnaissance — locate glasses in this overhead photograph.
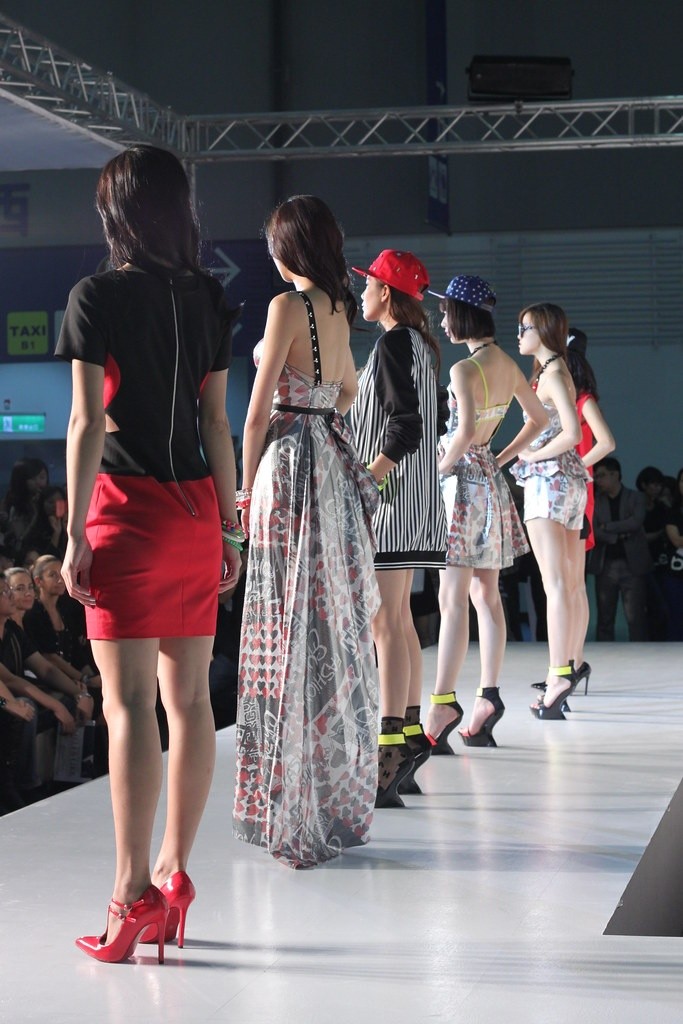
[0,586,13,598]
[518,322,537,338]
[592,472,613,482]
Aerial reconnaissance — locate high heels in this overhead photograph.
[530,670,580,720]
[138,872,196,948]
[75,884,168,964]
[398,723,432,795]
[529,659,592,695]
[422,691,464,756]
[459,686,505,747]
[373,733,416,808]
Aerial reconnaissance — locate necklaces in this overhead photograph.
[467,342,493,357]
[537,353,560,376]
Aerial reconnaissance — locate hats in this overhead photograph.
[428,274,497,313]
[351,249,431,302]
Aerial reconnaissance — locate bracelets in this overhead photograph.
[76,694,90,701]
[221,518,245,551]
[235,488,251,509]
[82,674,87,682]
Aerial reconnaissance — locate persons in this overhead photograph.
[426,275,549,755]
[410,390,547,648]
[54,143,241,965]
[509,303,616,718]
[209,542,249,703]
[349,250,449,809]
[592,457,683,641]
[0,458,107,815]
[230,194,383,871]
